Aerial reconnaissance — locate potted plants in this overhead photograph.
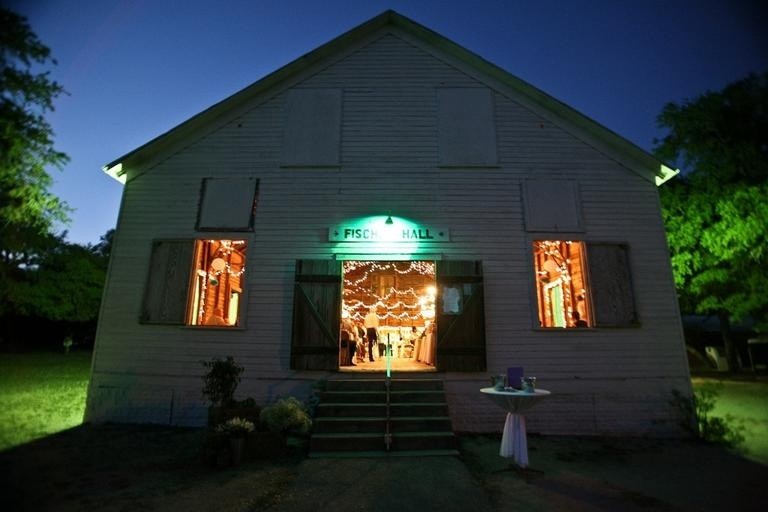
[198,356,260,429]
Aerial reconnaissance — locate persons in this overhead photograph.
[571,310,588,328]
[577,295,587,322]
[363,308,379,362]
[340,307,367,366]
[410,326,421,346]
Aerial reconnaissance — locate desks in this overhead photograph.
[479,387,551,478]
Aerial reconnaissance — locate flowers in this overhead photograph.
[213,418,254,434]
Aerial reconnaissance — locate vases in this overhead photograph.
[224,439,240,468]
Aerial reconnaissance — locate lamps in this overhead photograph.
[385,210,393,224]
[543,254,563,273]
[211,251,226,271]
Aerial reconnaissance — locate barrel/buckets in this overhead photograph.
[519,376,535,393]
